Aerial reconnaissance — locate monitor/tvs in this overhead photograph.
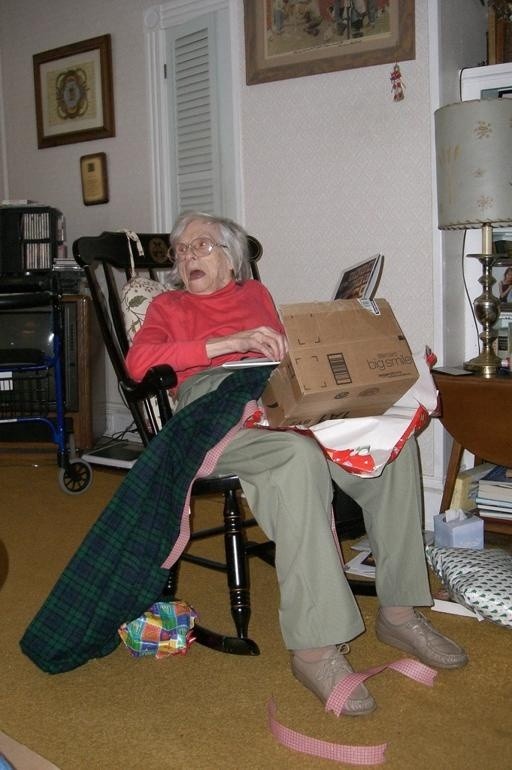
[0,302,78,412]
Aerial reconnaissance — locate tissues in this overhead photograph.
[432,506,486,550]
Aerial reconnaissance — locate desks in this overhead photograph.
[426,373,511,537]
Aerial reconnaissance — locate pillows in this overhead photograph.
[119,275,185,434]
[425,540,512,630]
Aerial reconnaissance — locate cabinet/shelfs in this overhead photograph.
[457,59,512,372]
[0,292,94,454]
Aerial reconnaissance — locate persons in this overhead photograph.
[496,268,511,302]
[125,209,473,719]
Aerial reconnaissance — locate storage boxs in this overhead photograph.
[246,297,421,428]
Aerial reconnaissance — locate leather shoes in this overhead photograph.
[377,609,467,668]
[293,652,377,716]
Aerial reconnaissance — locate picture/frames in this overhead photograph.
[331,252,384,303]
[28,33,117,152]
[244,2,416,86]
[486,258,511,312]
[79,152,110,208]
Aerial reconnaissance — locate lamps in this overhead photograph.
[434,94,511,379]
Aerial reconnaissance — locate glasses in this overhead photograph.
[167,238,227,262]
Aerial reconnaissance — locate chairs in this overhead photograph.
[72,222,381,655]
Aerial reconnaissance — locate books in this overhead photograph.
[449,461,512,521]
[24,212,52,271]
